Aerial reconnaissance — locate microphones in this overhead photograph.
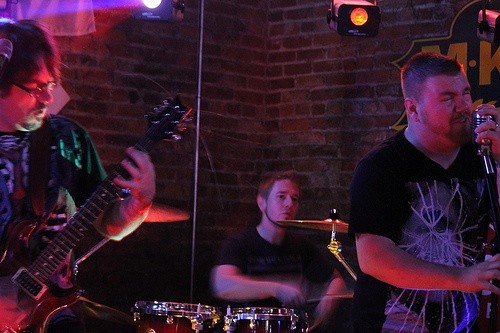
[473,108,495,144]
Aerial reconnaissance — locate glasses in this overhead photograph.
[12,79,57,98]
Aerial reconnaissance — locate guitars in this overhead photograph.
[0,93,194,333]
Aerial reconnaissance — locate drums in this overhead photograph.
[224,305,310,333]
[130,301,221,333]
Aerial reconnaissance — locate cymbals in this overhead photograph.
[144,202,191,223]
[277,219,349,233]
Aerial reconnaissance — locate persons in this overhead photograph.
[349,51,500,333]
[0,18,157,333]
[205,175,346,333]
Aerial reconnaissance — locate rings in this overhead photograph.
[496,122,499,130]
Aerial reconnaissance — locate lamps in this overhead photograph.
[132,0,185,22]
[327,0,382,38]
[476,0,500,45]
